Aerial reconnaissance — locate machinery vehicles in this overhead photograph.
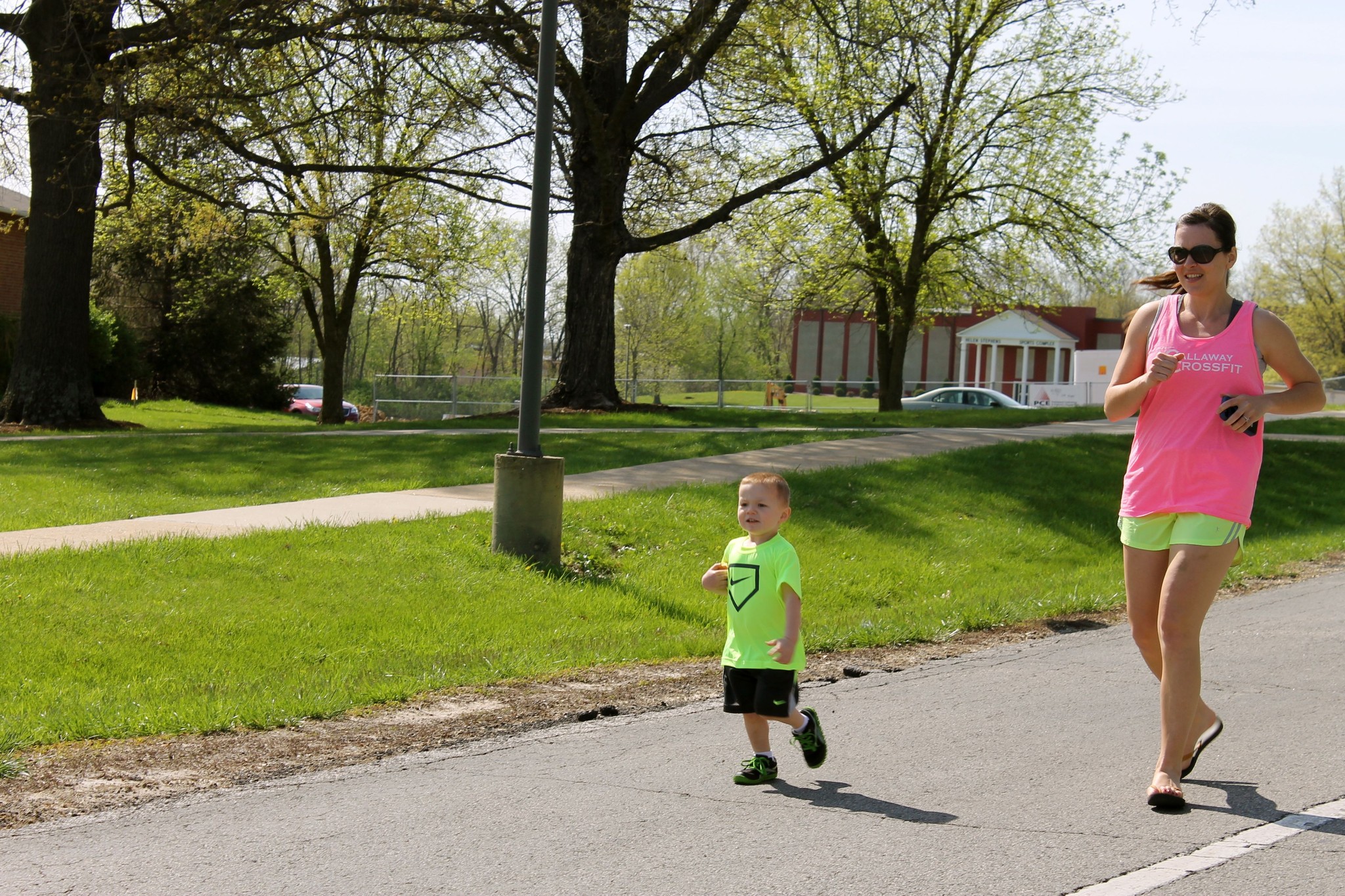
[764,381,787,407]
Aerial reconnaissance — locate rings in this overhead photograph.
[1243,416,1249,422]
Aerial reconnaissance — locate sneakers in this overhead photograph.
[732,754,780,785]
[789,706,827,769]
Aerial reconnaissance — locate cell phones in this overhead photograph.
[1219,396,1258,436]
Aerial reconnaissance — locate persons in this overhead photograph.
[701,473,826,782]
[1103,202,1325,803]
[968,396,975,404]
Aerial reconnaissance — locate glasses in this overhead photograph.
[1169,243,1224,264]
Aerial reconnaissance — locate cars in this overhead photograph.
[276,384,359,423]
[900,386,1038,412]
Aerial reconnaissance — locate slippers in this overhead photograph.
[1180,717,1223,780]
[1147,785,1186,806]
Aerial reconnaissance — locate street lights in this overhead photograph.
[623,324,631,401]
